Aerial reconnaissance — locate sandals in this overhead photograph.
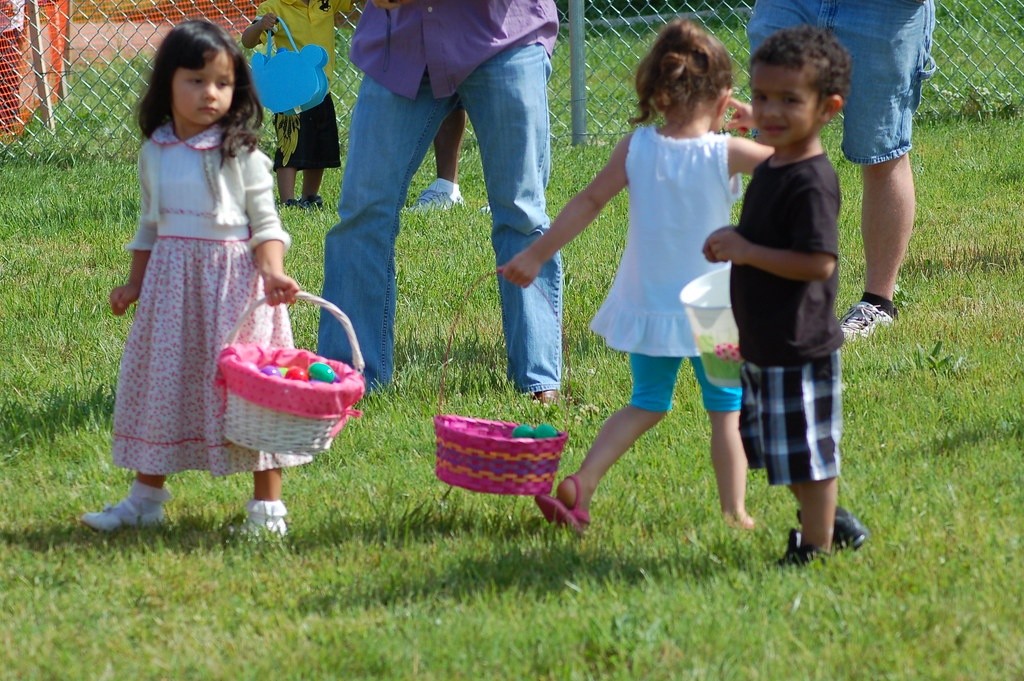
[532,476,590,538]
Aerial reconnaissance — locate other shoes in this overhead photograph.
[81,498,165,535]
[227,522,290,545]
[798,507,868,548]
[285,195,323,212]
[406,189,461,218]
[768,526,827,571]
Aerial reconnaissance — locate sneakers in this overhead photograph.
[834,303,895,344]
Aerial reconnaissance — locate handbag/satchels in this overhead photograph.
[251,17,330,117]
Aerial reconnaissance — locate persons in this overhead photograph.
[79,18,300,539]
[242,0,358,210]
[702,23,866,568]
[495,20,774,539]
[308,0,565,412]
[747,0,936,339]
[401,99,491,212]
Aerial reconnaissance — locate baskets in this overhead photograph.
[217,290,363,453]
[436,270,572,495]
